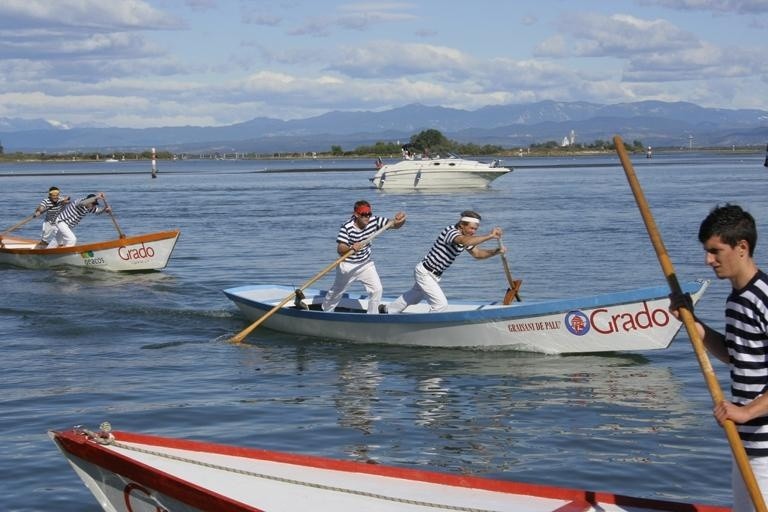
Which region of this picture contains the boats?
[47,421,734,512]
[368,147,514,188]
[0,229,180,272]
[223,279,709,356]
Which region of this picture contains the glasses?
[357,212,372,216]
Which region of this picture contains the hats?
[87,194,99,205]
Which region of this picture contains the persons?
[401,147,430,160]
[378,210,506,314]
[669,205,768,512]
[321,200,406,314]
[55,192,112,247]
[34,186,70,247]
